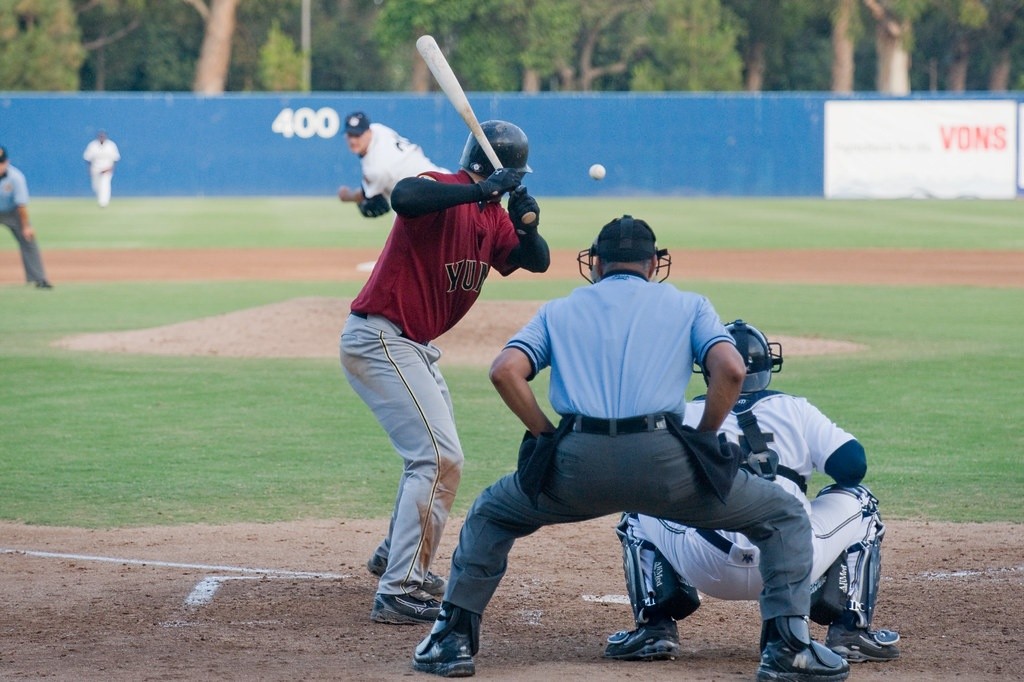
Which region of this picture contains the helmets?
[704,318,772,393]
[457,119,533,176]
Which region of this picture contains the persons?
[83,129,121,208]
[412,216,851,681]
[0,147,52,288]
[338,111,451,217]
[604,319,901,663]
[340,120,550,623]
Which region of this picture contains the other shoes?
[36,280,51,287]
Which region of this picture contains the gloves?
[480,166,521,202]
[505,187,541,244]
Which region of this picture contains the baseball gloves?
[357,193,390,217]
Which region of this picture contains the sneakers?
[367,552,449,595]
[757,616,850,680]
[603,612,679,660]
[371,590,441,623]
[825,622,900,661]
[412,602,482,677]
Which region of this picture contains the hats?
[345,111,370,137]
[595,214,656,264]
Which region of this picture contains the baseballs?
[588,163,606,180]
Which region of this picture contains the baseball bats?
[415,34,537,224]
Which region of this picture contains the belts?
[351,311,429,347]
[696,527,733,554]
[571,415,667,435]
